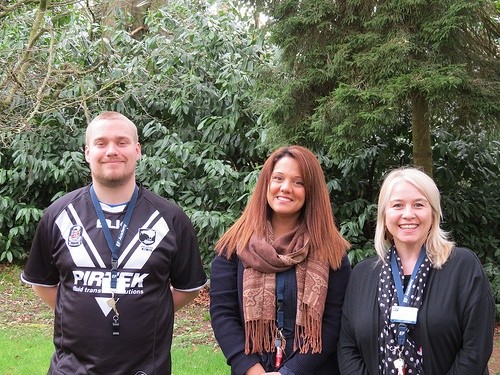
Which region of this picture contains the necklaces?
[90,184,139,336]
[276,272,286,368]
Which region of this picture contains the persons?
[337,167,494,375]
[20,111,207,375]
[211,146,351,375]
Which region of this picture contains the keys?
[107,298,119,316]
[393,357,405,375]
[275,338,282,354]
